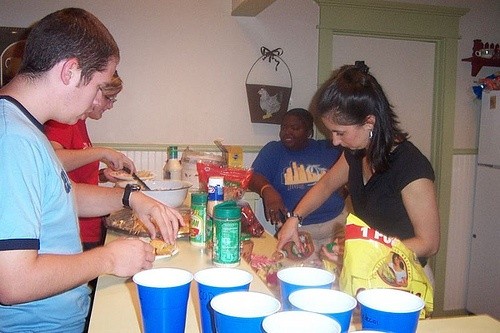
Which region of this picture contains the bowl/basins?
[114,179,193,207]
[106,168,156,181]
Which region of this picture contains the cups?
[261,311,343,333]
[357,288,425,333]
[277,267,335,311]
[132,267,193,333]
[194,267,253,333]
[209,290,281,333]
[288,288,358,333]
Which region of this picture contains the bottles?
[207,175,225,222]
[212,201,243,266]
[273,231,315,263]
[190,192,209,247]
[239,233,254,264]
[163,146,181,180]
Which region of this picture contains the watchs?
[123,183,141,211]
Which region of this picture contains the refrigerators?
[466,87,500,323]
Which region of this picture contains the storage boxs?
[214,140,243,168]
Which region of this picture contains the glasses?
[100,88,117,103]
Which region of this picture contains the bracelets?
[260,184,272,198]
[99,168,109,183]
[285,212,304,228]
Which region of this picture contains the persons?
[0,7,184,333]
[44,70,137,289]
[247,107,348,260]
[276,61,441,298]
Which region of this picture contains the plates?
[126,237,179,258]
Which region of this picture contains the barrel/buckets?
[179,151,224,193]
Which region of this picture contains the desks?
[88,189,500,333]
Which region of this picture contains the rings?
[271,209,274,214]
